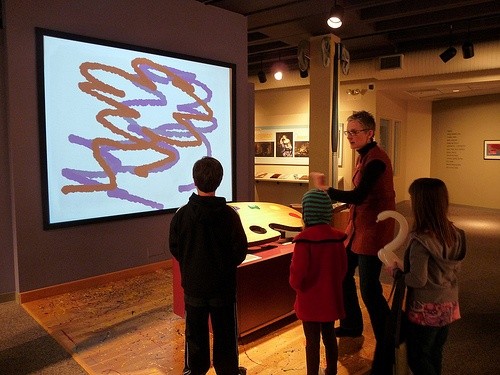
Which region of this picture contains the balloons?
[376,210,409,271]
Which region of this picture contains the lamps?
[326,1,344,29]
[462,22,475,59]
[439,25,457,63]
[257,57,268,83]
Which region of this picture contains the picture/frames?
[484,140,500,160]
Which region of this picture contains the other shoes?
[238,366,247,375]
[335,325,363,336]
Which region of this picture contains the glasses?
[343,128,369,136]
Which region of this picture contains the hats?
[301,188,334,224]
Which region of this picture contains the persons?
[309,110,395,341]
[169,157,248,375]
[383,178,466,375]
[289,189,348,375]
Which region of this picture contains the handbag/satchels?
[393,342,409,375]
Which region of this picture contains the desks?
[172,237,296,338]
